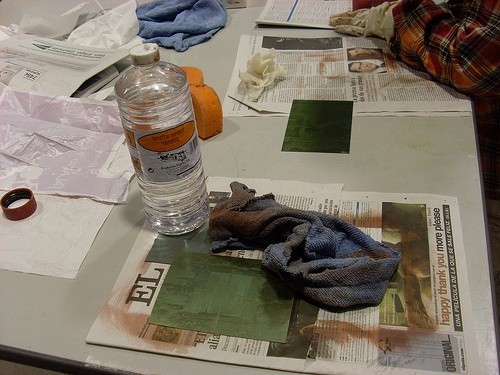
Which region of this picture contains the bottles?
[115,43,210,235]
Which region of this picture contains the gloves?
[328,0,398,45]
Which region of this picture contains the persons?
[347,48,383,59]
[347,59,389,75]
[329,0,500,323]
